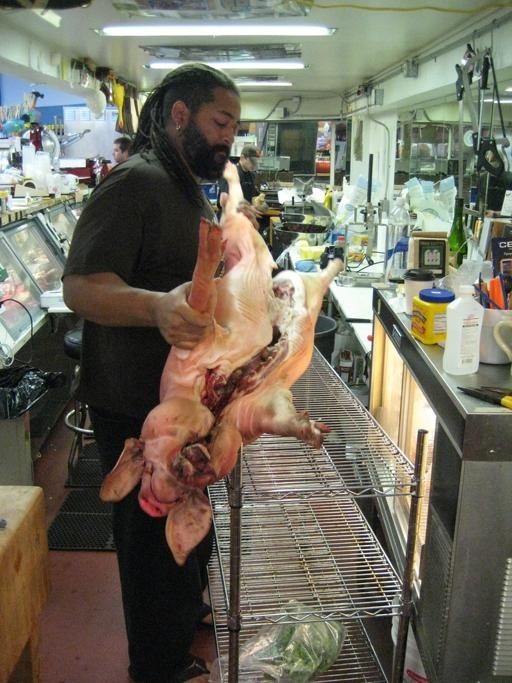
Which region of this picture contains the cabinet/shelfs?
[204,343,428,681]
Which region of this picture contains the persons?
[110,135,135,161]
[218,144,262,225]
[61,62,253,683]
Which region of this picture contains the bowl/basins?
[273,221,330,247]
[259,211,306,224]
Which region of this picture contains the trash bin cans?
[265,194,278,200]
[266,202,284,212]
[274,222,326,251]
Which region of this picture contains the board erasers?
[345,319,372,323]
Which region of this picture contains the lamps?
[88,19,333,86]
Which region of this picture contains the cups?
[481,308,510,364]
[401,267,434,316]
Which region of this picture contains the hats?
[242,144,262,163]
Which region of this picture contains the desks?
[323,272,374,409]
[0,483,54,682]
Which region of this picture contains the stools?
[60,326,102,487]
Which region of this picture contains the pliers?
[481,386,512,395]
[457,386,512,410]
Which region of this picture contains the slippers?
[128,657,211,683]
[197,603,214,627]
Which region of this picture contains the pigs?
[98,159,344,566]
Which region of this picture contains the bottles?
[444,284,481,371]
[388,197,408,282]
[412,288,452,345]
[445,197,469,270]
[468,186,479,209]
[334,235,345,244]
